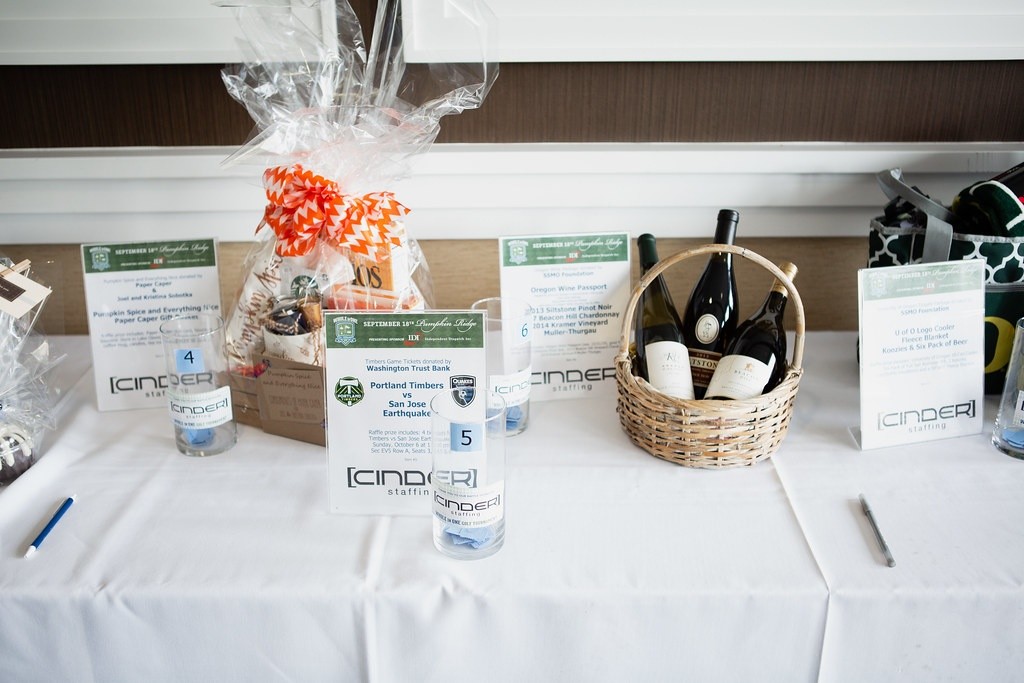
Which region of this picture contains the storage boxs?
[213,344,327,448]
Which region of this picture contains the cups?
[990,317,1024,461]
[159,312,238,458]
[428,387,507,560]
[472,296,534,438]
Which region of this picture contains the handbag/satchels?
[857,163,1023,395]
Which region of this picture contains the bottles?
[683,209,740,401]
[637,233,696,402]
[703,260,799,401]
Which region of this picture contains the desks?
[0,333,1023,683]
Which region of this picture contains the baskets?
[613,245,805,468]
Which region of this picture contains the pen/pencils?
[857,493,897,570]
[24,493,78,561]
